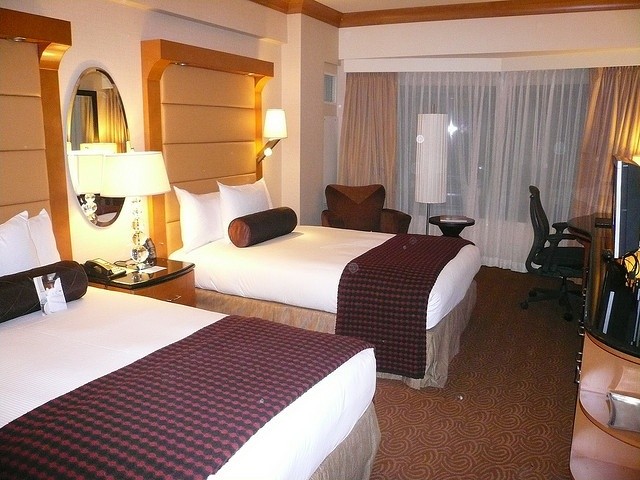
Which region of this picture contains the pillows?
[28,209,61,266]
[228,206,297,248]
[0,260,88,324]
[173,186,222,254]
[216,179,275,244]
[1,211,40,278]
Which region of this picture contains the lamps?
[257,108,289,164]
[79,141,118,153]
[416,115,447,236]
[69,150,113,230]
[97,147,174,280]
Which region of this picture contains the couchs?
[322,184,411,234]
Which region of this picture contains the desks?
[567,213,640,480]
[428,215,476,237]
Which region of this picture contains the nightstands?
[89,259,195,310]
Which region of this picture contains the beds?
[0,7,381,479]
[140,39,482,391]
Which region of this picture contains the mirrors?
[64,67,131,228]
[70,89,97,149]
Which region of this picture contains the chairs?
[520,186,591,321]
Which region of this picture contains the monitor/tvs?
[611,153,640,259]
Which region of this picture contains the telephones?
[80,258,126,290]
